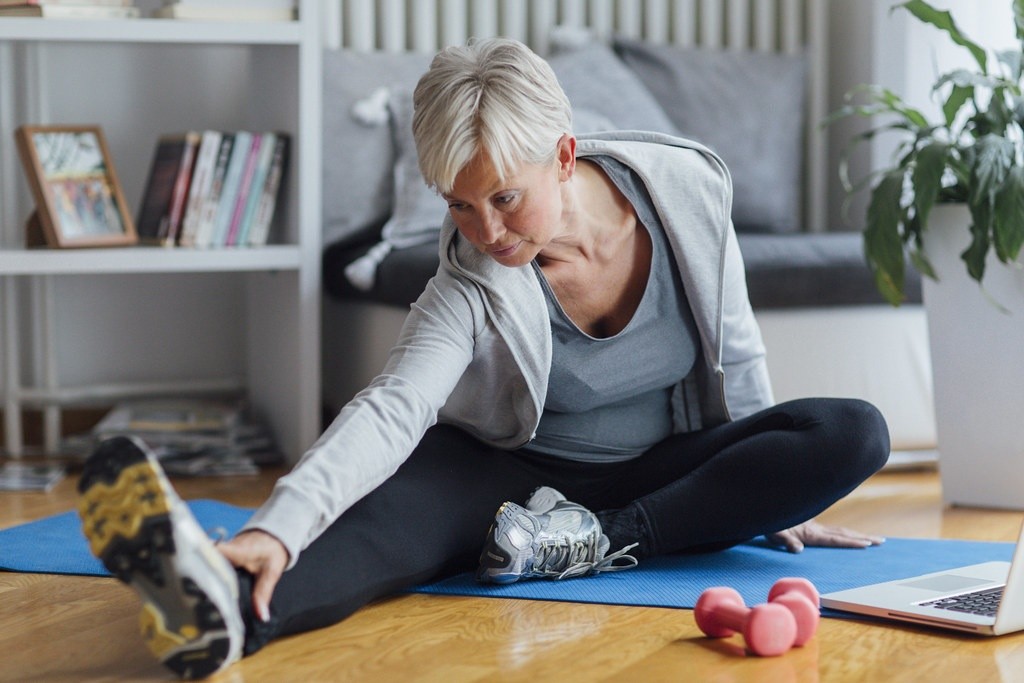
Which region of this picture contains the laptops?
[818,521,1024,635]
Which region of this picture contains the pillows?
[344,83,608,291]
[618,33,806,230]
[545,27,685,146]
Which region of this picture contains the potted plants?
[820,0,1024,510]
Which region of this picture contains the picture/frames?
[15,122,137,249]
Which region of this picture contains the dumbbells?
[693,586,798,656]
[768,576,819,647]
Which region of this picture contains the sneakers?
[77,431,249,683]
[476,483,641,585]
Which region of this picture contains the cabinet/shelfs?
[0,18,321,466]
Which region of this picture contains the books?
[136,130,289,248]
[98,397,274,476]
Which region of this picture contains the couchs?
[320,41,946,473]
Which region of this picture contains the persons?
[77,44,890,683]
